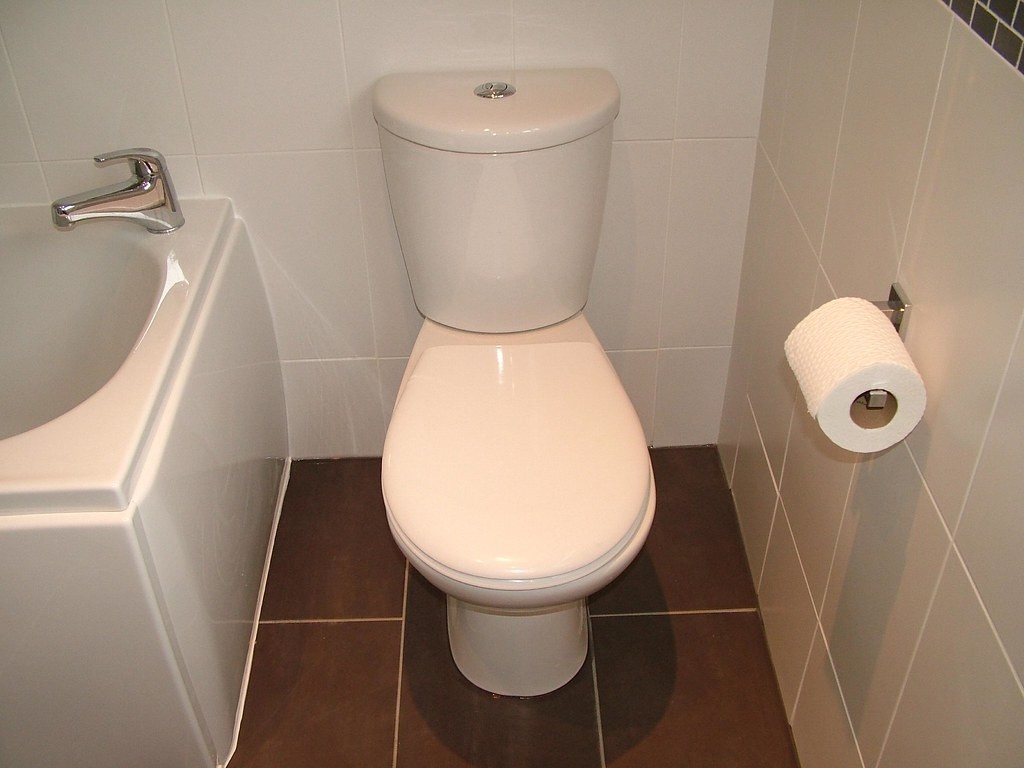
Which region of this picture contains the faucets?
[50,147,184,235]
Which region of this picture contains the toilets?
[373,67,658,697]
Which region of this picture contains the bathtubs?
[0,195,290,767]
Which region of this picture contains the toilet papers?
[783,295,927,454]
